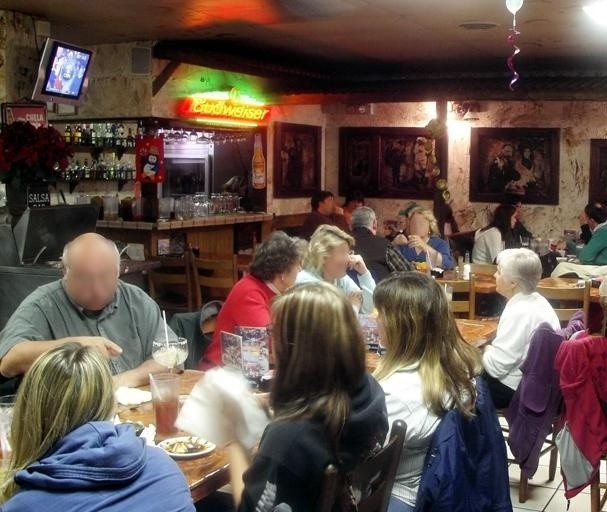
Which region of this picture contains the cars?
[158,192,241,221]
[148,374,181,435]
[518,233,547,253]
[440,284,453,308]
[74,195,139,222]
[0,394,17,473]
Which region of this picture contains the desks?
[23,251,161,289]
[537,277,599,309]
[3,314,500,512]
[436,264,499,293]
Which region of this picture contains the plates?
[45,141,138,185]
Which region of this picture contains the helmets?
[156,436,216,459]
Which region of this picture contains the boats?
[130,45,152,76]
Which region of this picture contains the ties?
[31,38,96,107]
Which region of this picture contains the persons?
[481,247,560,411]
[303,194,453,282]
[472,198,535,317]
[371,272,485,510]
[487,143,552,194]
[295,224,377,319]
[0,232,185,392]
[578,202,605,265]
[413,137,431,183]
[0,341,197,512]
[203,230,301,369]
[290,237,309,268]
[228,282,389,510]
[391,146,403,185]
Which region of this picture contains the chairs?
[495,311,585,503]
[535,280,590,329]
[436,273,475,319]
[148,250,191,319]
[191,253,238,311]
[562,336,606,512]
[446,228,605,275]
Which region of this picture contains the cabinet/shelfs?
[250,134,266,190]
[462,251,471,281]
[63,125,135,148]
[61,160,132,181]
[454,266,460,280]
[45,141,138,185]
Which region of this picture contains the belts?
[159,127,246,147]
[151,337,187,374]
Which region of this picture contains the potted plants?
[271,118,323,200]
[588,137,607,207]
[468,125,562,207]
[337,124,449,200]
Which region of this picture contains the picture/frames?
[588,137,607,207]
[337,124,449,200]
[271,118,323,200]
[468,125,562,207]
